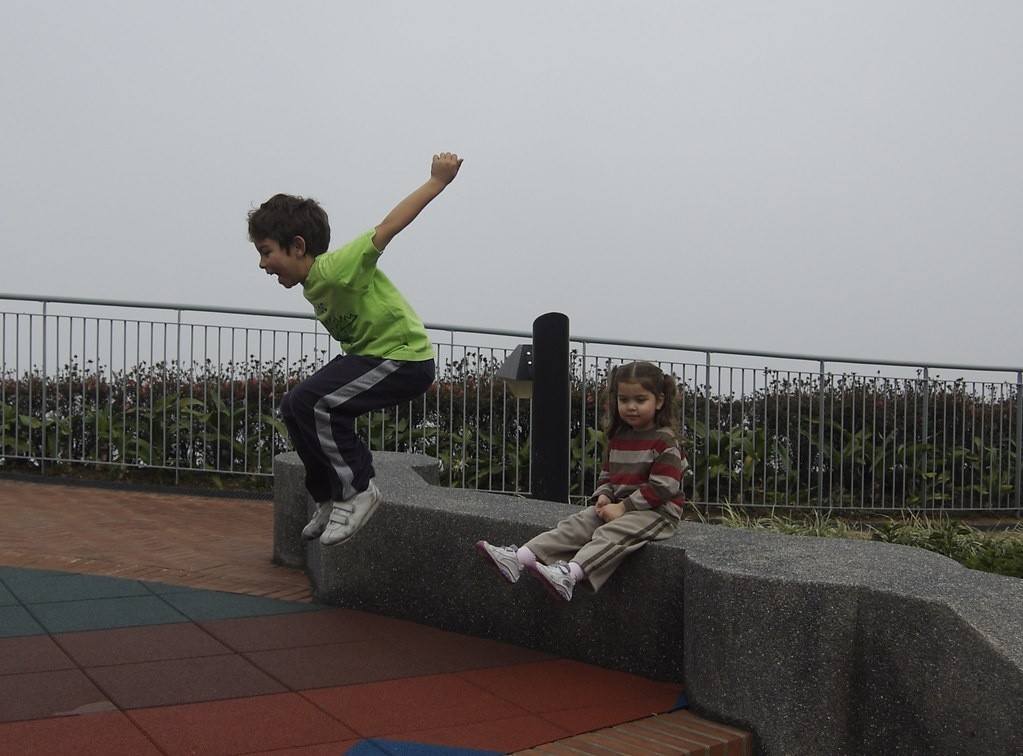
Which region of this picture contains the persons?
[475,361,694,603]
[246,152,464,549]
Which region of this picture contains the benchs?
[271,450,1023,756]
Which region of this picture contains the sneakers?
[526,560,576,602]
[320,478,383,548]
[476,540,525,583]
[301,500,333,540]
